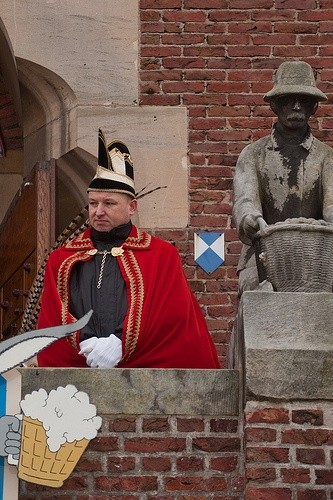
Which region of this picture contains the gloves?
[78,334,122,368]
[77,337,98,357]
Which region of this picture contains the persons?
[233,61,333,292]
[15,129,222,369]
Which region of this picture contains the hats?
[86,128,139,201]
